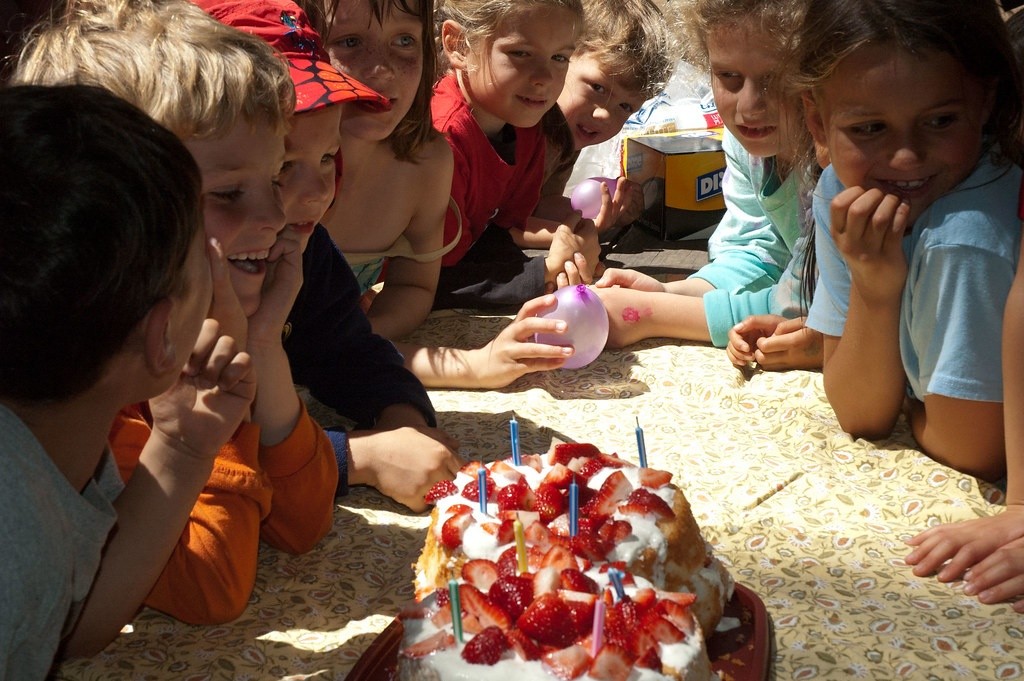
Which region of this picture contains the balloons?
[571,177,617,219]
[536,284,610,370]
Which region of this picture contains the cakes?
[397,438,731,681]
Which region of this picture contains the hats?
[191,0,392,115]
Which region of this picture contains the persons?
[0,0,670,681]
[551,0,815,348]
[722,0,1024,483]
[903,256,1024,615]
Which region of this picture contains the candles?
[448,570,463,645]
[568,475,580,536]
[510,410,519,465]
[478,460,488,511]
[591,588,606,653]
[514,513,528,572]
[635,415,648,467]
[604,557,623,597]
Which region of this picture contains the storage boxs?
[622,127,728,242]
